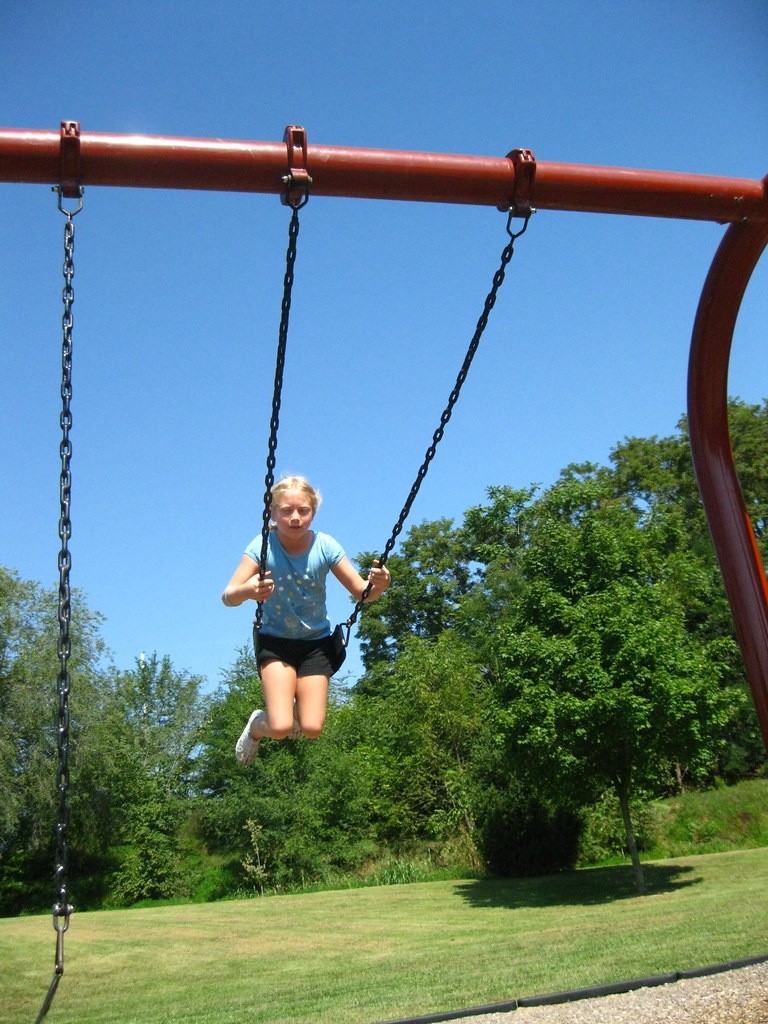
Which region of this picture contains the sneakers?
[289,697,302,734]
[236,710,266,768]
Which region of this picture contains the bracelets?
[224,590,242,607]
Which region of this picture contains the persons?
[222,475,390,766]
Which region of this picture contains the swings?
[244,120,544,683]
[30,115,87,1024]
